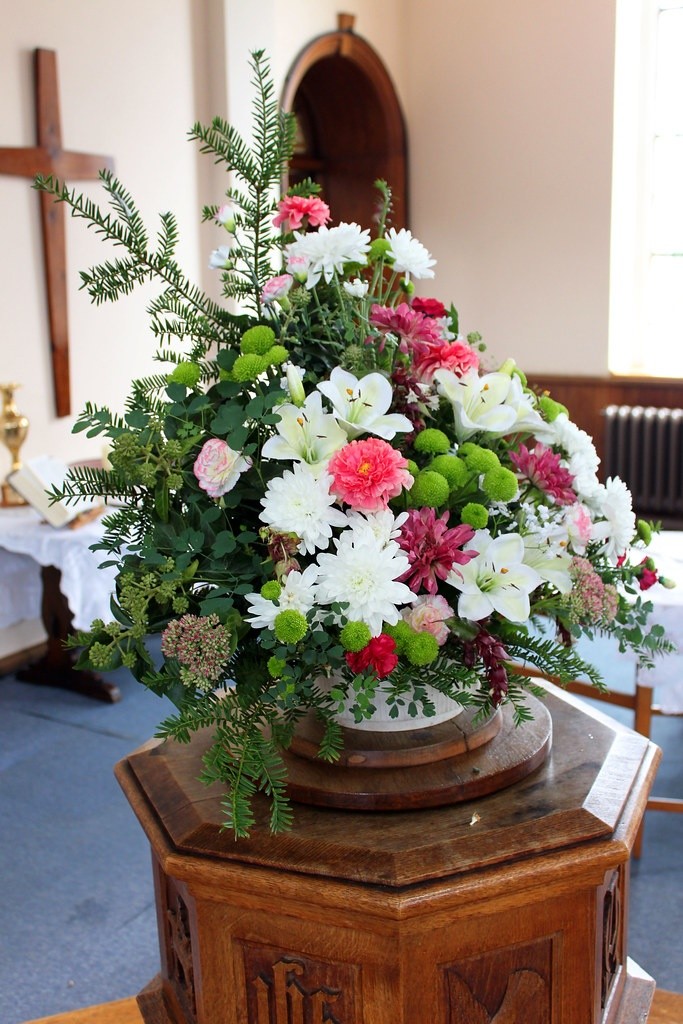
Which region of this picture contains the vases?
[331,661,471,732]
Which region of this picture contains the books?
[6,452,99,531]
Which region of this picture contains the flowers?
[30,44,673,842]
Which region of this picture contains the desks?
[0,499,140,705]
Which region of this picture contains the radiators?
[606,405,683,521]
